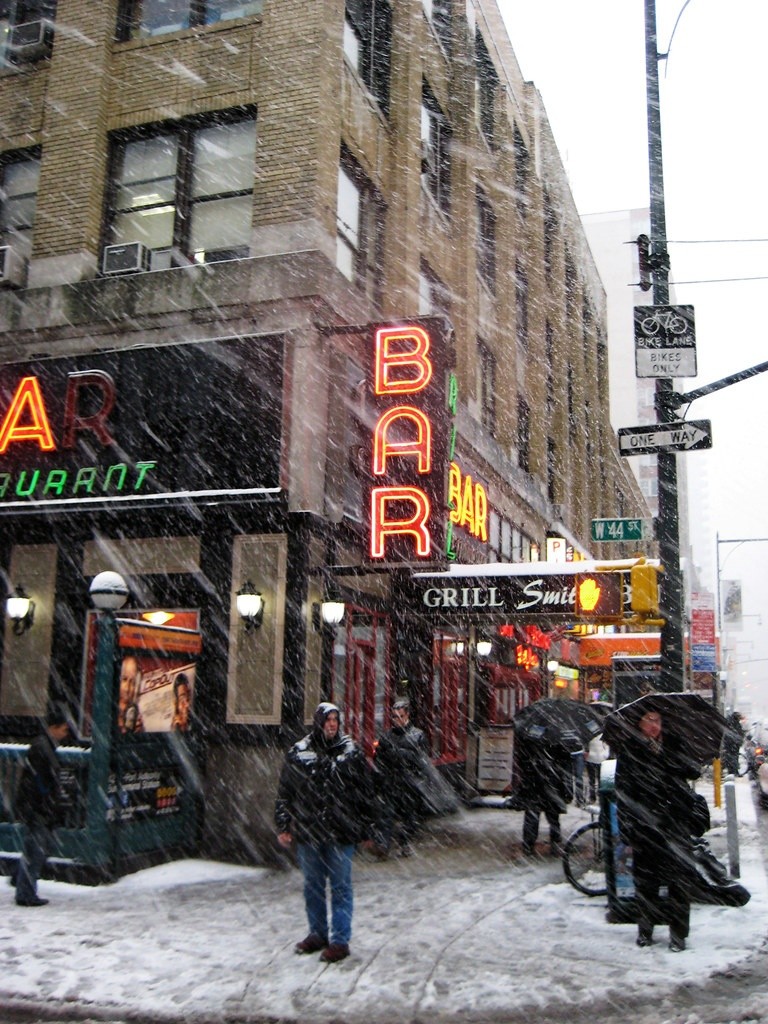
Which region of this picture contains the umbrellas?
[589,700,616,715]
[505,698,605,744]
[599,690,729,778]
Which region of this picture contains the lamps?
[476,634,491,661]
[545,656,559,676]
[237,579,263,632]
[88,568,129,622]
[320,588,345,635]
[6,584,32,636]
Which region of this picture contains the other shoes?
[637,930,652,944]
[17,898,48,906]
[667,937,685,951]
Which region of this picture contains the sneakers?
[296,934,329,954]
[320,944,348,962]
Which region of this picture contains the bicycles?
[562,804,631,896]
[739,753,750,777]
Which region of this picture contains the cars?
[742,717,768,810]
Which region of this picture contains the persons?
[118,656,145,734]
[586,734,610,804]
[614,705,694,952]
[10,711,67,907]
[510,732,574,858]
[725,711,746,777]
[368,702,426,862]
[171,673,192,731]
[274,702,372,963]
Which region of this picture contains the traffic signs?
[618,418,713,457]
[590,519,643,543]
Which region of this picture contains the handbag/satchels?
[690,796,711,836]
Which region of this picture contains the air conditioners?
[101,242,151,277]
[9,19,54,56]
[0,244,27,290]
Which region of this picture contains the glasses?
[393,714,406,719]
